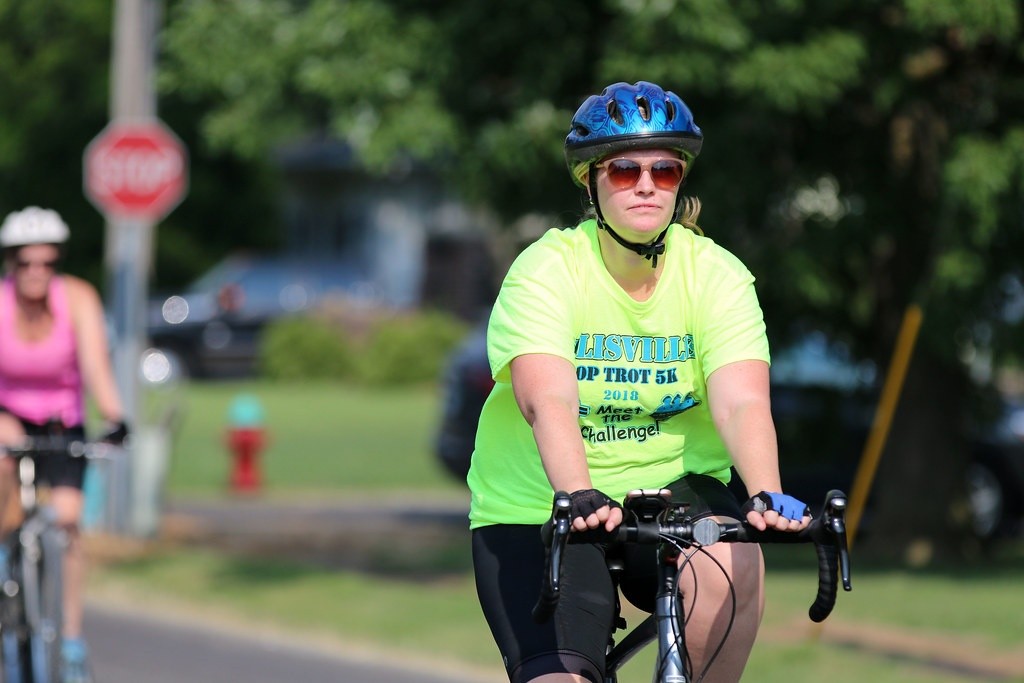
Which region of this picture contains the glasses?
[13,259,59,270]
[597,157,687,192]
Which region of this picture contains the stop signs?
[82,121,189,225]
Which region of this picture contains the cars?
[107,255,405,381]
[433,300,1023,548]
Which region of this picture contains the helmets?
[0,206,71,249]
[564,81,704,189]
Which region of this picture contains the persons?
[464,81,815,683]
[0,204,139,683]
[431,287,500,483]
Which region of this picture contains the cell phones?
[626,489,672,503]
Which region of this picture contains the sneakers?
[59,637,92,683]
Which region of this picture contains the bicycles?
[1,414,130,682]
[530,488,851,683]
